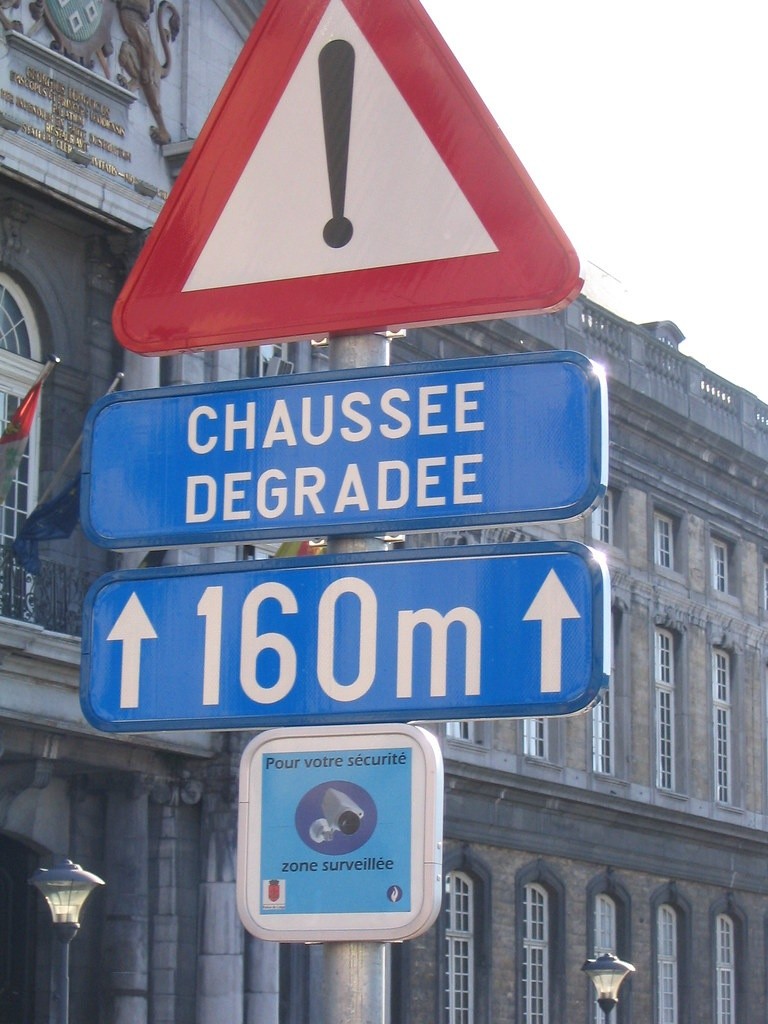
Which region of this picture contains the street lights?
[581,953,636,1024]
[26,855,110,1024]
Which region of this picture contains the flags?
[0,380,48,508]
[11,470,82,574]
